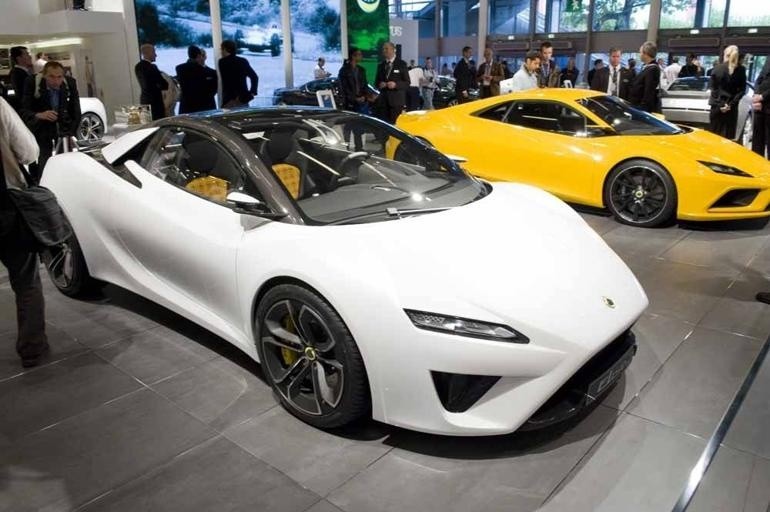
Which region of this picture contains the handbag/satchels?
[7,181,72,249]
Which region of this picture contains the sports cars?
[232,22,296,58]
[20,104,649,438]
[381,87,770,227]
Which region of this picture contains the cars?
[75,98,109,147]
[408,76,480,111]
[660,75,754,151]
[272,78,379,109]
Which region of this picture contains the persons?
[1,46,81,182]
[588,42,770,159]
[0,93,49,369]
[407,40,579,115]
[315,42,410,155]
[134,41,259,119]
[756,292,770,304]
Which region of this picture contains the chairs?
[168,128,238,199]
[253,119,316,195]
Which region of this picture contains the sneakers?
[15,336,49,368]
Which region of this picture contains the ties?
[384,60,393,78]
[609,67,620,99]
[486,66,490,75]
